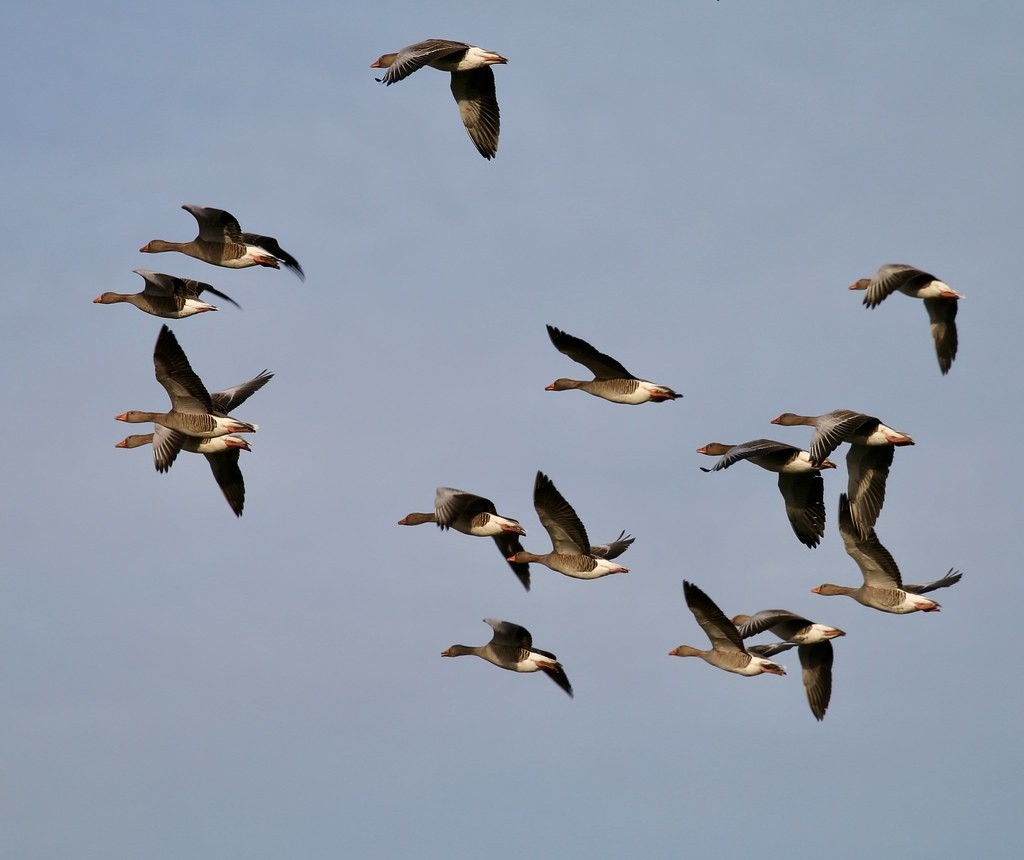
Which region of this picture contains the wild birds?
[667,580,846,723]
[695,408,915,550]
[441,616,573,698]
[397,470,636,592]
[811,493,963,615]
[545,324,684,406]
[847,264,966,377]
[93,205,309,516]
[370,38,510,161]
